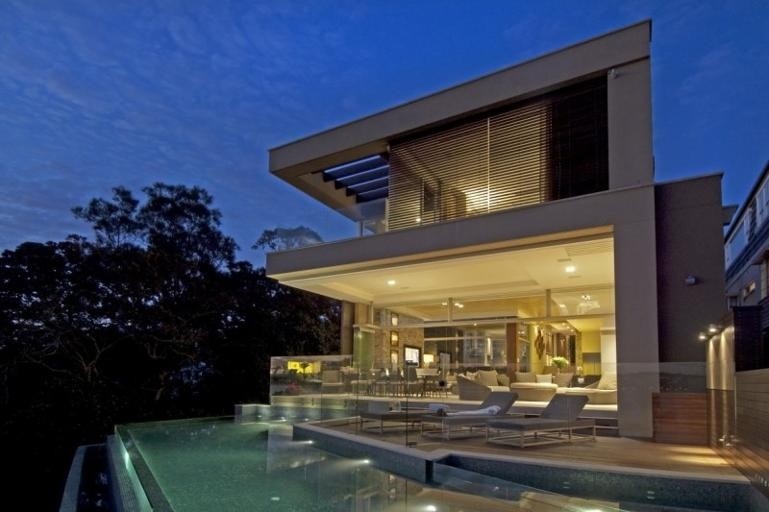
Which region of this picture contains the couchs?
[457,371,512,399]
[510,371,574,400]
[556,385,617,403]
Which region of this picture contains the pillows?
[515,371,536,382]
[497,374,510,385]
[595,371,617,390]
[535,374,553,384]
[476,370,499,387]
[465,371,477,380]
[553,373,573,386]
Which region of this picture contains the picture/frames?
[390,349,399,365]
[390,331,399,347]
[390,311,400,328]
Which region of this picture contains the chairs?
[483,393,597,448]
[366,367,448,398]
[420,392,524,441]
[361,407,456,434]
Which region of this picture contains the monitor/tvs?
[403,344,422,366]
